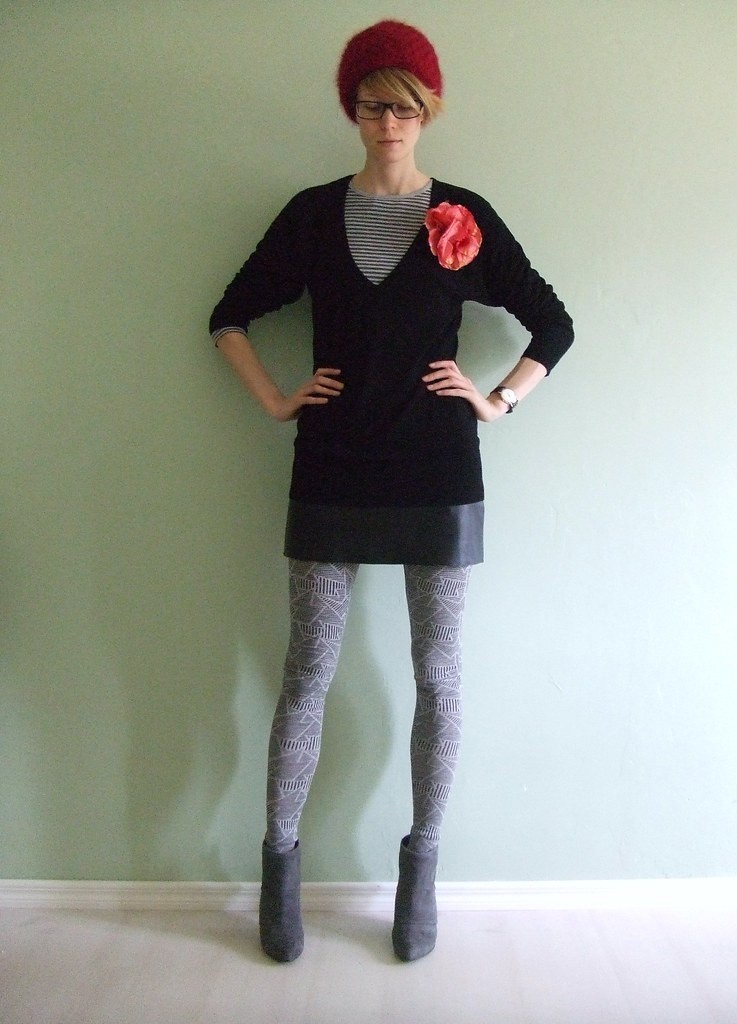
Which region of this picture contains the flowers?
[423,201,483,270]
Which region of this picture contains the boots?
[392,835,438,961]
[259,838,305,962]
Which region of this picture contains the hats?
[336,21,441,122]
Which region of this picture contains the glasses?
[354,100,422,120]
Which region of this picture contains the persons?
[211,20,574,962]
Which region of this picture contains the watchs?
[489,386,519,414]
